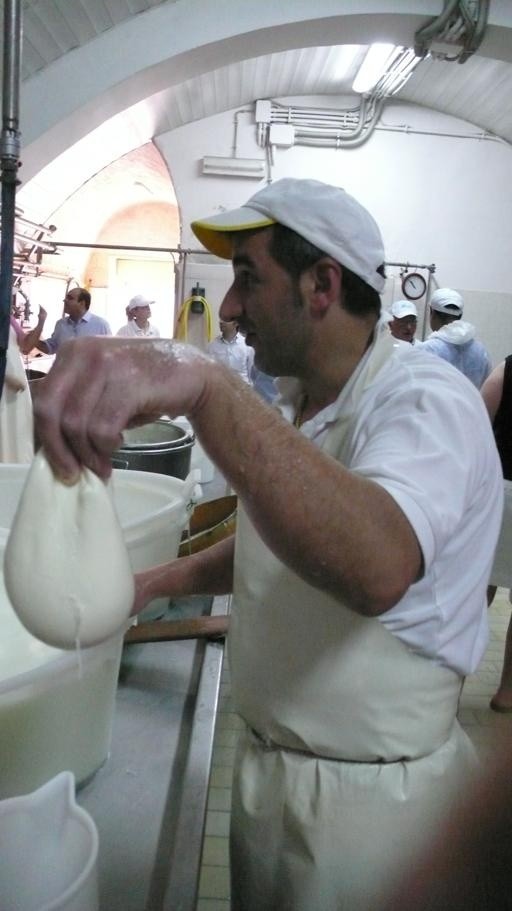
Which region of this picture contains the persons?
[8,286,159,356]
[388,286,510,714]
[33,178,512,910]
[210,320,279,401]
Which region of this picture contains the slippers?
[490,699,510,713]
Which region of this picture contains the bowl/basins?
[116,418,195,480]
[2,463,195,805]
[180,495,239,554]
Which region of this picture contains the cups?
[2,772,102,910]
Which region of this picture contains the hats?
[128,296,155,312]
[191,179,386,294]
[431,287,464,316]
[391,300,418,319]
